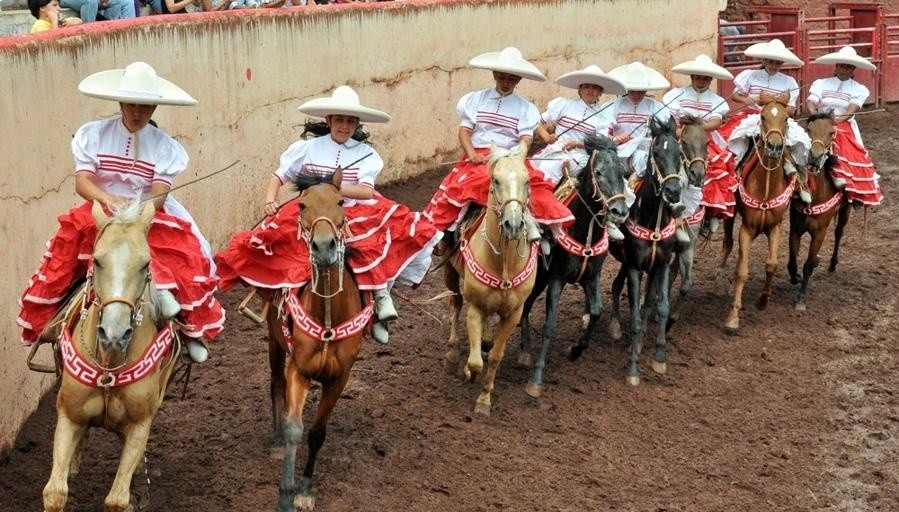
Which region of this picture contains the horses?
[581,115,682,389]
[442,137,540,416]
[786,109,851,310]
[696,89,795,335]
[643,113,712,340]
[516,134,630,397]
[264,164,366,512]
[42,197,176,512]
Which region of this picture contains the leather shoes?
[607,221,625,242]
[832,176,847,188]
[371,295,399,345]
[524,211,551,256]
[709,216,720,234]
[157,287,209,364]
[784,162,812,204]
[671,202,690,243]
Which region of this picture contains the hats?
[77,61,198,106]
[607,62,670,91]
[554,65,627,95]
[743,38,804,66]
[815,46,877,71]
[670,53,734,79]
[298,85,392,124]
[470,47,546,82]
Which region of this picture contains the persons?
[526,63,627,242]
[600,60,693,246]
[803,43,886,213]
[662,53,739,234]
[14,58,228,366]
[210,85,446,344]
[719,37,814,209]
[418,45,576,265]
[24,0,399,40]
